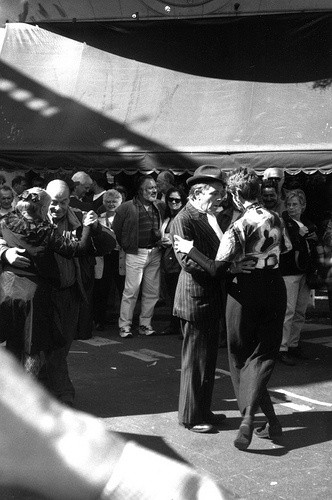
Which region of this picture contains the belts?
[138,243,158,249]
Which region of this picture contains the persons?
[113,177,163,339]
[171,164,256,432]
[261,182,282,211]
[281,186,319,365]
[174,168,291,450]
[0,169,187,406]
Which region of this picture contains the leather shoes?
[205,413,226,422]
[255,423,282,440]
[187,423,213,433]
[234,425,251,451]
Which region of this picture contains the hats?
[186,164,228,186]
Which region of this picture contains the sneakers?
[119,328,132,338]
[140,328,155,335]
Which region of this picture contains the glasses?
[268,177,285,182]
[167,196,183,203]
